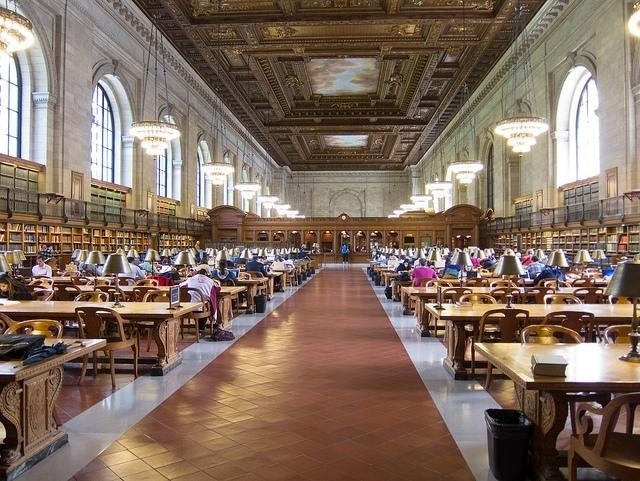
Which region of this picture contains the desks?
[473,343,640,481]
[0,338,107,481]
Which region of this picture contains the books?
[492,222,639,257]
[0,220,195,254]
[532,354,568,378]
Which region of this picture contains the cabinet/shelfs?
[490,218,640,269]
[0,218,202,256]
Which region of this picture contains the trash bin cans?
[296,266,316,285]
[366,265,383,285]
[253,295,267,314]
[484,409,533,481]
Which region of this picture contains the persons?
[0,274,32,301]
[370,245,616,288]
[32,256,52,278]
[341,241,350,264]
[76,241,293,339]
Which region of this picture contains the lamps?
[257,93,306,219]
[130,0,182,156]
[425,30,453,198]
[0,0,35,53]
[387,88,432,218]
[235,55,261,199]
[494,0,550,153]
[201,0,234,186]
[451,0,484,185]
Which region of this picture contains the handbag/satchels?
[0,334,45,361]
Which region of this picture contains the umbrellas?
[12,338,86,369]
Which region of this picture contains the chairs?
[521,323,611,435]
[370,246,640,389]
[567,392,640,481]
[3,318,64,426]
